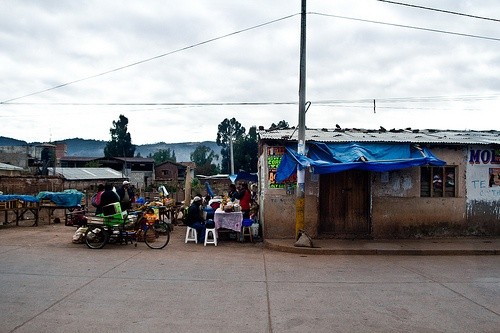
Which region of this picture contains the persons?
[92,181,255,243]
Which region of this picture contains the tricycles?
[84,209,170,250]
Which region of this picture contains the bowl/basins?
[240,219,253,227]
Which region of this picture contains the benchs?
[65,211,84,225]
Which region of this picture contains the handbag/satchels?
[294,231,314,247]
[205,219,215,229]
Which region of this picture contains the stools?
[185,226,197,244]
[241,226,253,243]
[204,227,217,247]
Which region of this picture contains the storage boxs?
[243,219,252,226]
[103,210,129,227]
[102,202,122,216]
[5,193,86,209]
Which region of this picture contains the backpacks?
[92,191,105,207]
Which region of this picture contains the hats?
[194,196,201,201]
[123,181,130,185]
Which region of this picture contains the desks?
[158,209,178,227]
[0,206,39,228]
[214,208,244,242]
[40,206,85,226]
